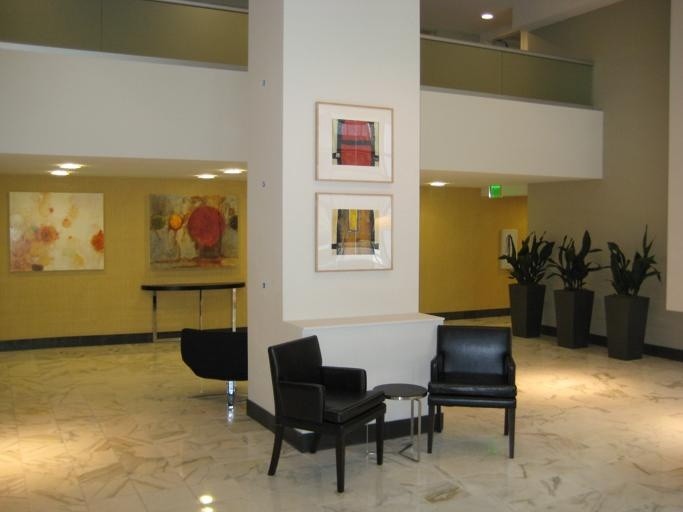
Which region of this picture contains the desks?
[142,282,245,344]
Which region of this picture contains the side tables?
[367,383,427,462]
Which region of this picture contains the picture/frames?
[315,192,393,272]
[315,102,394,183]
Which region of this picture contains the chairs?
[428,325,517,459]
[268,335,387,493]
[180,328,247,406]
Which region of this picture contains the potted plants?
[497,230,555,338]
[603,224,661,360]
[546,230,603,348]
